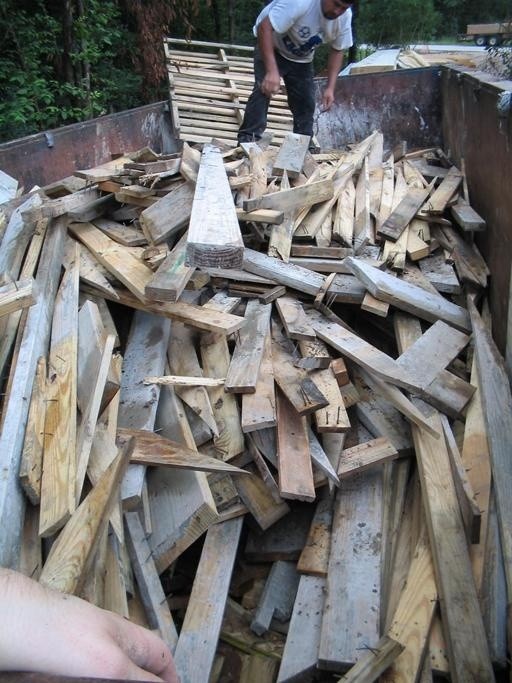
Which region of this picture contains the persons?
[1,561,183,682]
[237,0,355,146]
